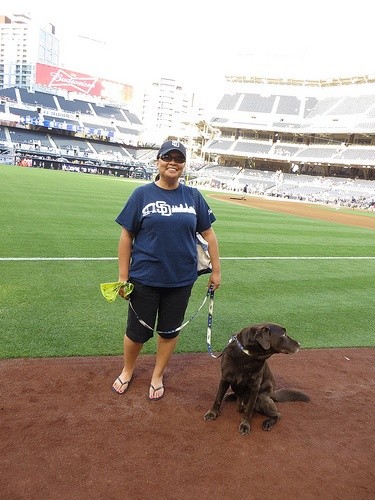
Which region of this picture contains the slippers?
[149,383,164,401]
[112,376,128,395]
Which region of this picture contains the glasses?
[158,154,186,164]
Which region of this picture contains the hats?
[157,140,187,161]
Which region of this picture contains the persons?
[91,134,109,141]
[333,196,375,212]
[111,140,221,402]
[109,170,111,176]
[241,184,248,200]
[99,169,104,175]
[115,171,118,176]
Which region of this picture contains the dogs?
[204,322,310,437]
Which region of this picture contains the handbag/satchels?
[195,231,212,275]
